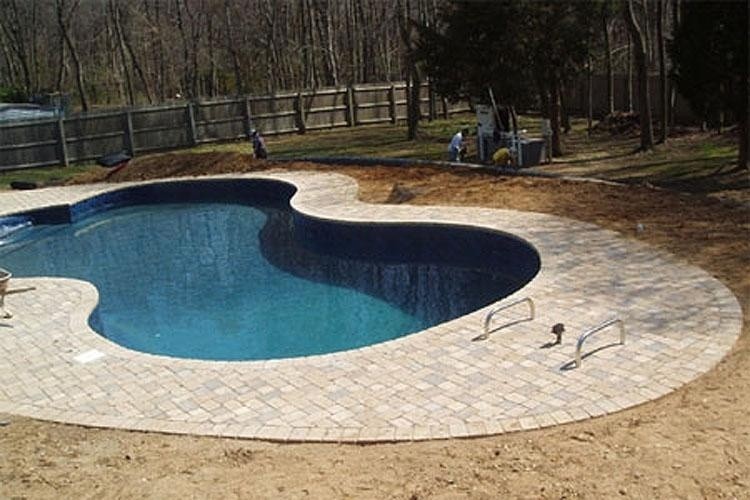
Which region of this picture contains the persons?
[250,130,267,160]
[541,111,554,163]
[448,128,468,162]
[491,146,518,182]
